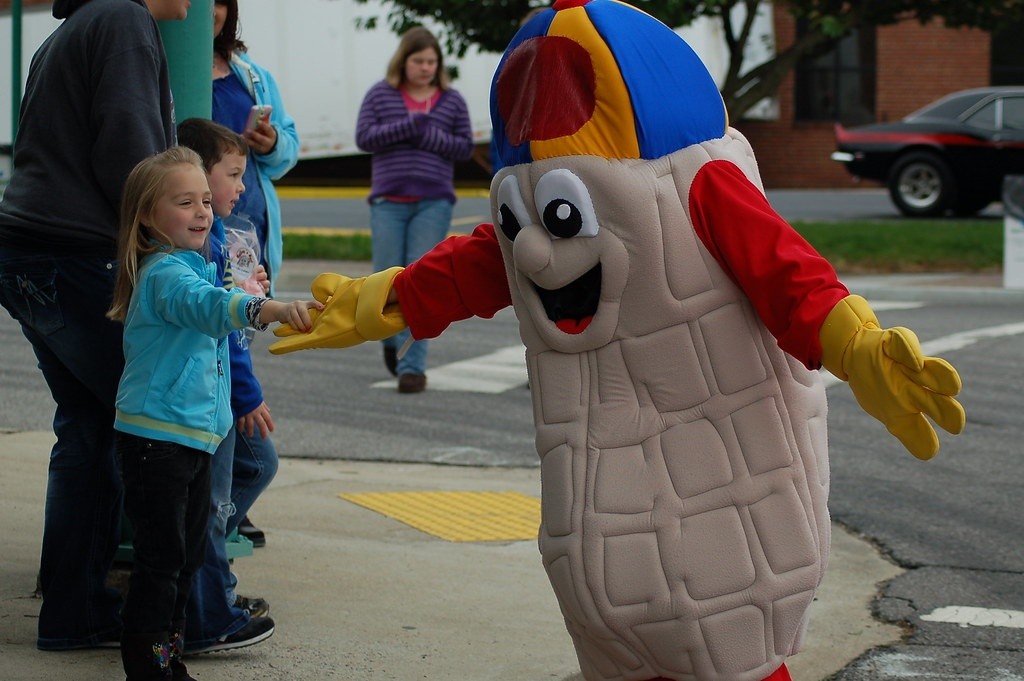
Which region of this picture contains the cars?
[831,88,1024,219]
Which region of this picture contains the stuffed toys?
[268,0,966,681]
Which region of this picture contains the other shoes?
[397,373,426,393]
[238,519,268,547]
[384,346,398,376]
[234,593,269,617]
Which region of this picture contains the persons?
[106,142,324,681]
[177,117,275,653]
[354,28,475,394]
[211,0,301,302]
[1,0,274,654]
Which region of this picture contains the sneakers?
[178,614,277,656]
[38,601,122,651]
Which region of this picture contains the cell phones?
[244,104,273,133]
